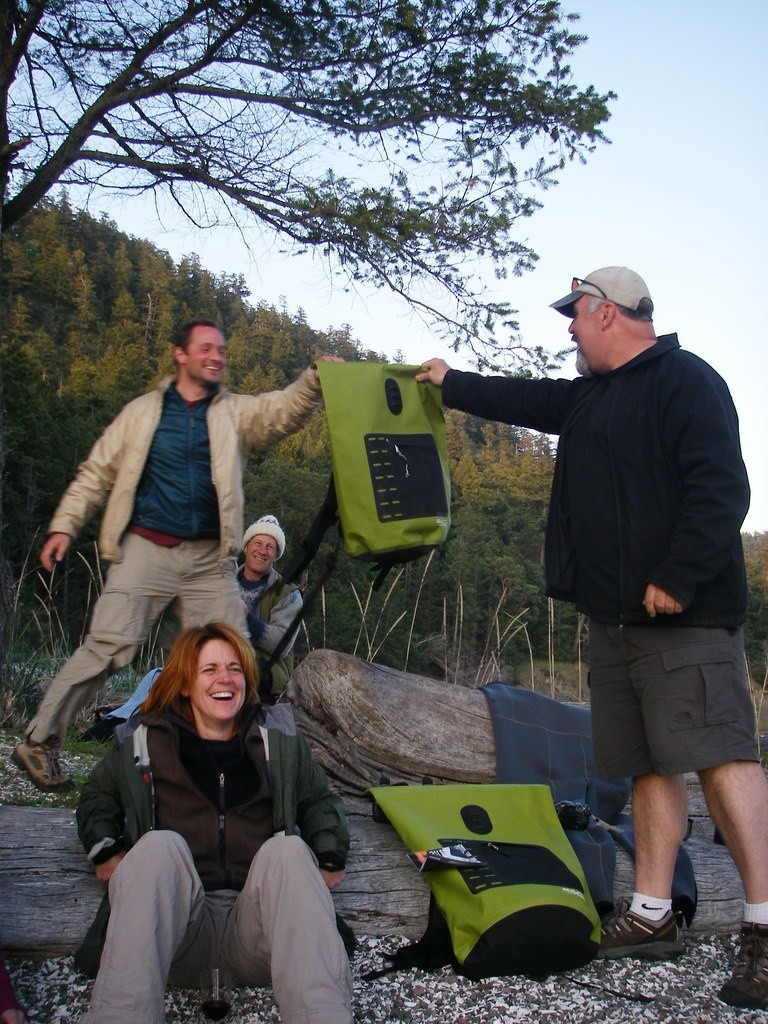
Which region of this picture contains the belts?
[127,524,183,548]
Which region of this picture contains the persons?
[11,321,347,792]
[416,267,768,1011]
[77,622,358,1024]
[0,950,30,1024]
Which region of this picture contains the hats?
[242,514,286,561]
[548,266,654,318]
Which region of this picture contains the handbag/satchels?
[308,359,453,561]
[362,783,603,978]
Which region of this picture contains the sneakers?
[596,895,687,961]
[11,740,75,793]
[718,921,768,1011]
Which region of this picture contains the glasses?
[571,277,606,299]
